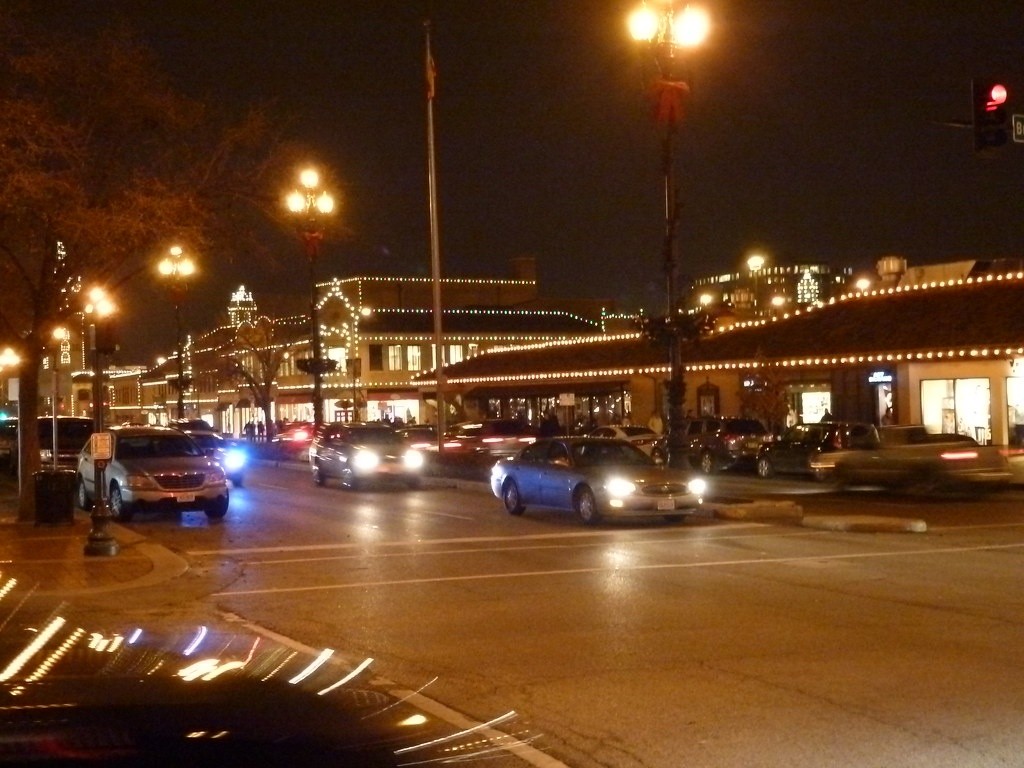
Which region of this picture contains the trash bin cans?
[31,470,76,527]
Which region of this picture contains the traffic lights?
[971,69,1009,152]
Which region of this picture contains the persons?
[378,414,417,429]
[882,408,895,425]
[244,418,297,445]
[541,410,694,436]
[821,409,833,422]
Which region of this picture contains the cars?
[387,422,441,454]
[806,423,1008,506]
[0,417,19,463]
[490,438,704,526]
[439,418,540,465]
[271,421,340,462]
[76,427,231,522]
[586,426,659,465]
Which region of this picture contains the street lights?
[74,281,114,432]
[160,245,199,419]
[40,323,67,425]
[0,346,20,419]
[280,166,338,483]
[625,3,708,479]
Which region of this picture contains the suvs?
[165,419,226,465]
[310,421,421,491]
[652,415,771,477]
[753,417,878,487]
[35,417,93,471]
